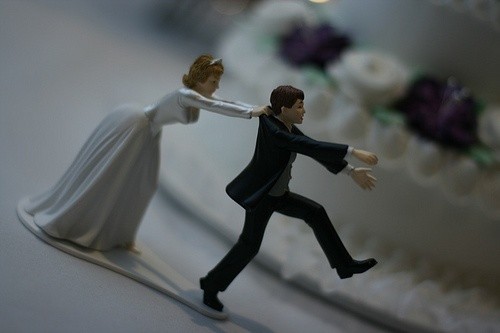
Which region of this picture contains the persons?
[199,85,378,312]
[25,53,275,255]
